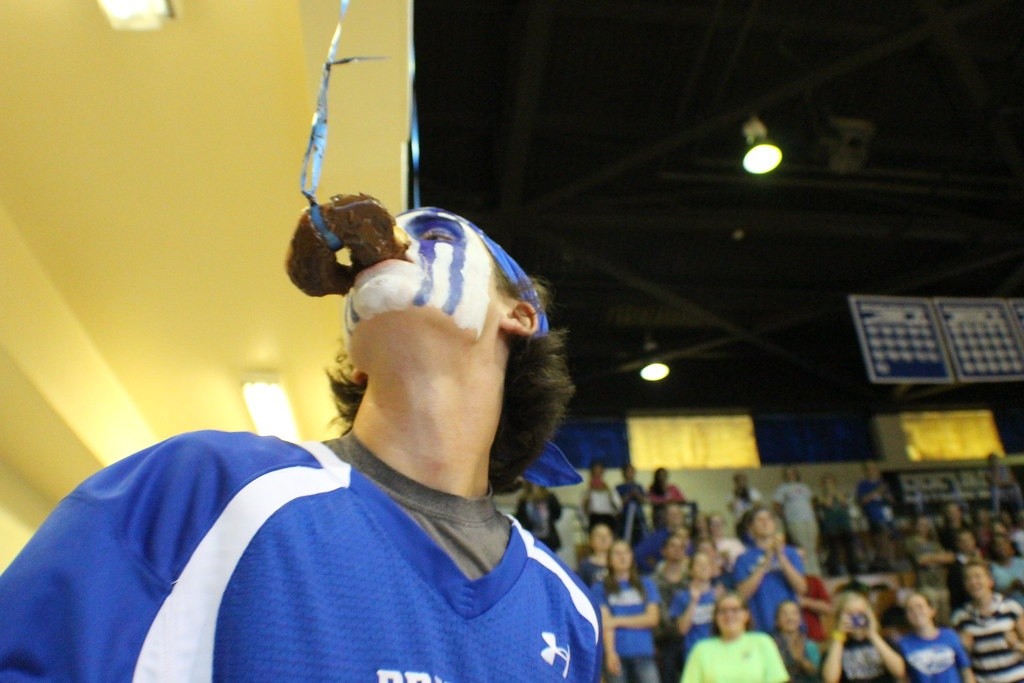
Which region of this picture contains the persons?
[509,452,1023,683]
[1,205,610,681]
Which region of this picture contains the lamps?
[639,342,670,382]
[741,116,783,176]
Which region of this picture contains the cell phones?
[848,615,868,626]
